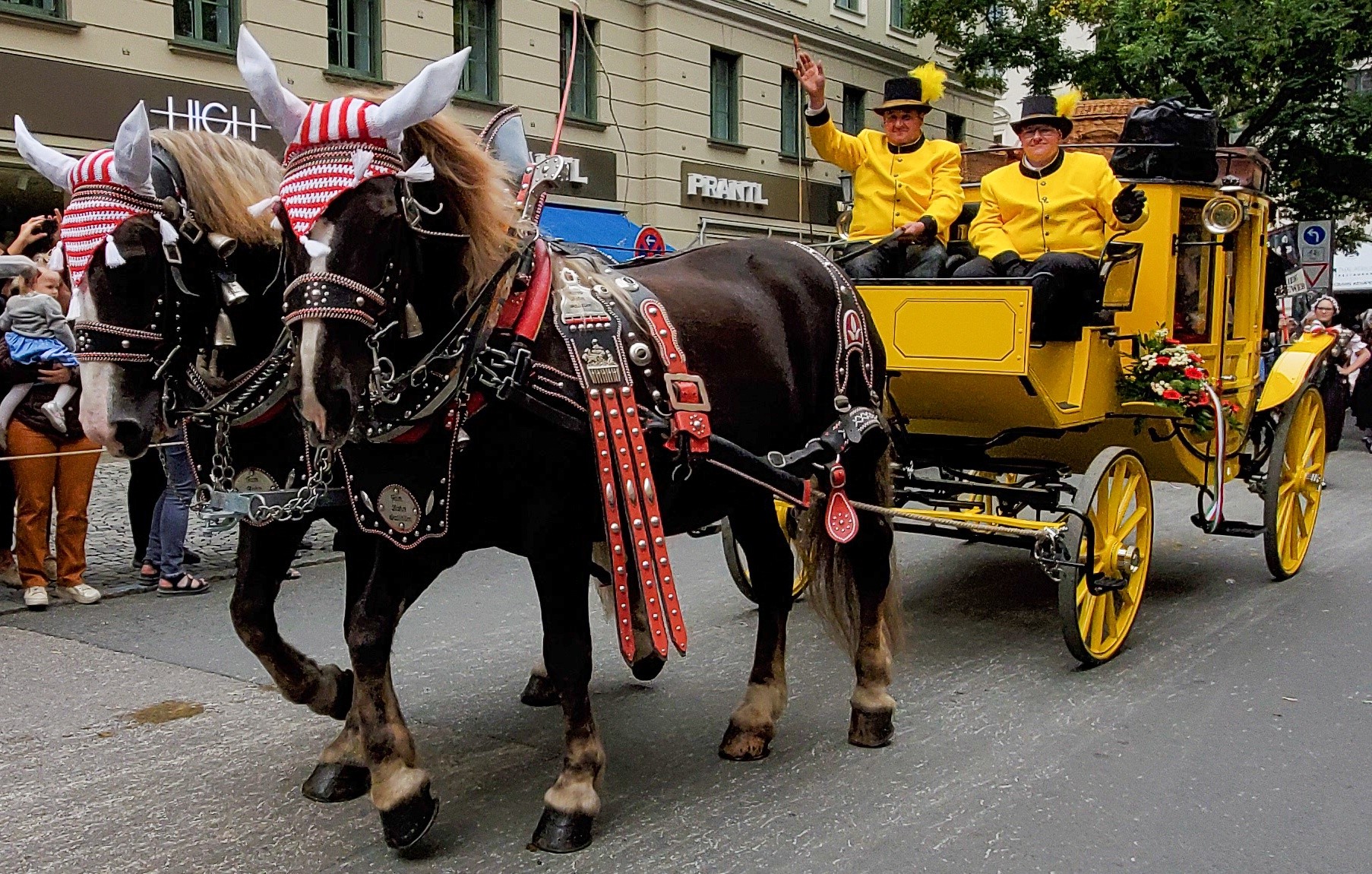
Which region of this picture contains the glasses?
[1316,306,1333,313]
[1018,126,1060,138]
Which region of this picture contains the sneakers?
[24,585,49,606]
[55,582,101,604]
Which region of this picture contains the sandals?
[155,570,209,597]
[136,560,160,586]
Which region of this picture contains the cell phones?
[41,220,58,233]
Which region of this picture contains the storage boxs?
[959,147,1007,183]
[1215,147,1271,193]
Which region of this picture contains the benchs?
[821,202,1123,418]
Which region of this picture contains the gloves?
[1114,182,1147,215]
[1002,259,1031,278]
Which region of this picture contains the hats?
[874,62,948,116]
[1310,295,1340,315]
[1010,90,1083,139]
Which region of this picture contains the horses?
[11,22,912,853]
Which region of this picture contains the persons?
[950,96,1149,338]
[1266,295,1372,488]
[791,50,966,284]
[0,210,314,608]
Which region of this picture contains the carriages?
[16,24,1334,851]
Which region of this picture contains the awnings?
[537,205,678,264]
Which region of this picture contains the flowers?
[1114,319,1250,437]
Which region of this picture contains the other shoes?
[0,423,8,450]
[0,550,23,585]
[1321,479,1327,489]
[132,550,144,567]
[182,545,201,564]
[1361,436,1372,454]
[42,553,59,581]
[41,401,67,433]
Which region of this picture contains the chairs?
[1173,271,1212,343]
